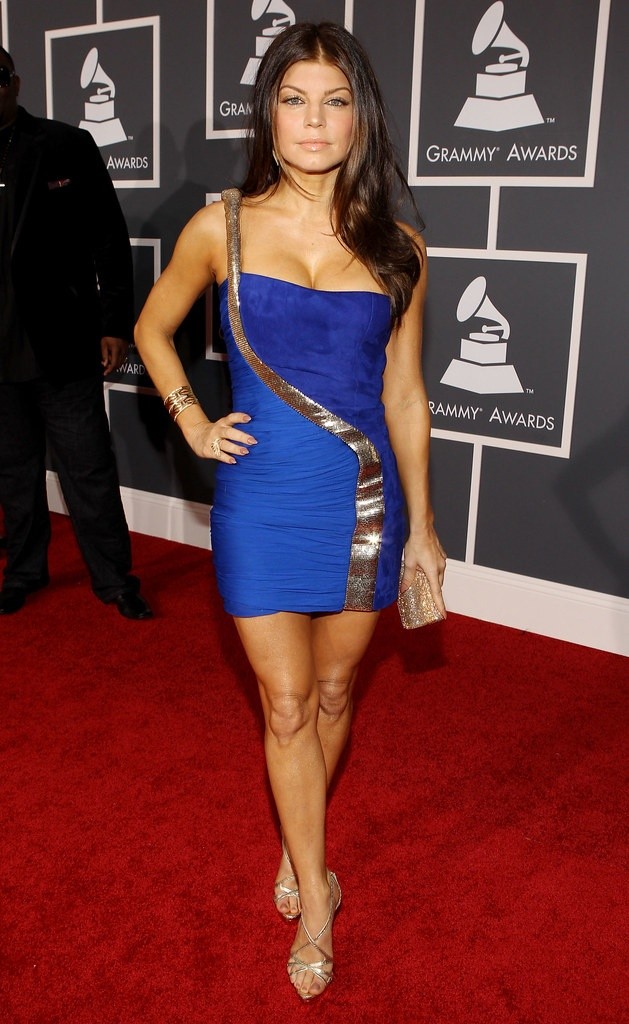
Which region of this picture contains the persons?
[0,44,152,620]
[134,22,448,1000]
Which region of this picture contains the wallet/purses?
[397,547,443,628]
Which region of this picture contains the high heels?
[287,870,342,1001]
[274,844,303,919]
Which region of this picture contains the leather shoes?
[95,577,153,620]
[0,574,47,614]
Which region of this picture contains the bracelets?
[163,385,199,423]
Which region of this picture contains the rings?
[211,438,225,457]
